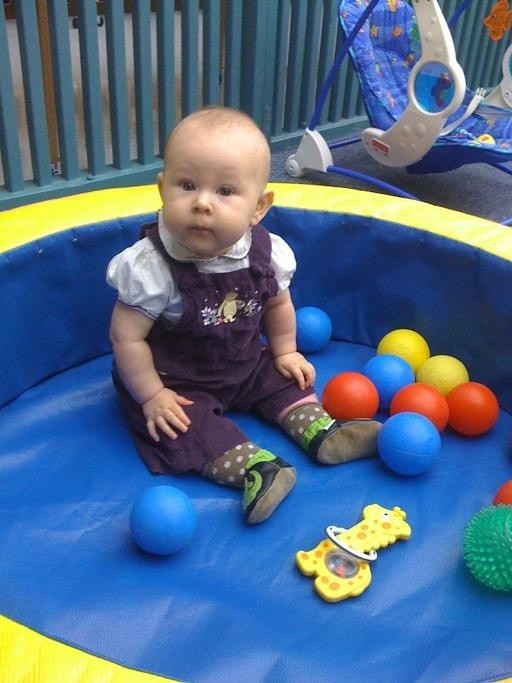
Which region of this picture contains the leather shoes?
[242,450,297,524]
[302,416,383,465]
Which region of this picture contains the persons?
[105,107,383,525]
